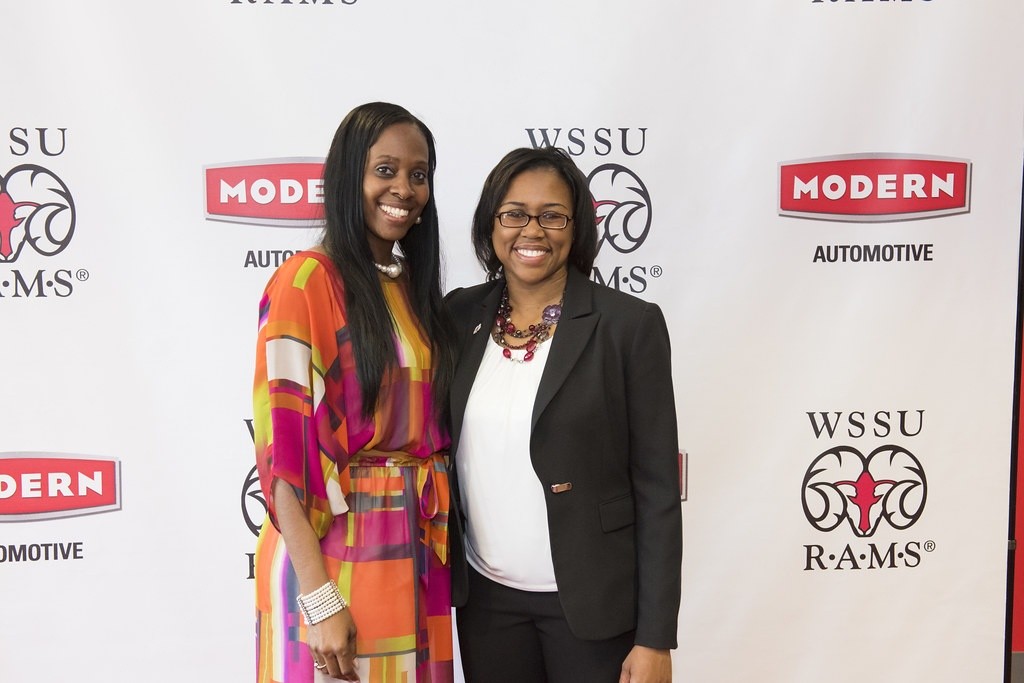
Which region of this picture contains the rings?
[313,660,326,669]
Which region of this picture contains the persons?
[438,142,685,683]
[247,98,456,682]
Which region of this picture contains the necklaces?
[367,252,403,279]
[496,284,564,364]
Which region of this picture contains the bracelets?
[296,577,348,626]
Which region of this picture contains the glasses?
[494,212,574,229]
